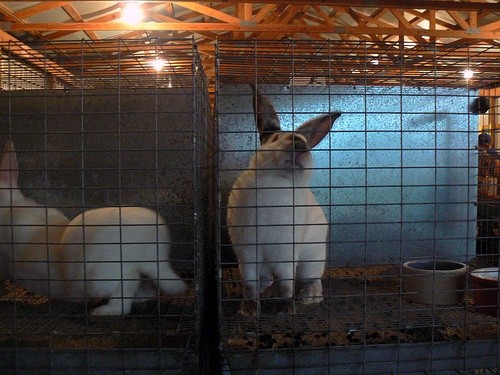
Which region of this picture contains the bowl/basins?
[403,260,467,304]
[470,267,500,316]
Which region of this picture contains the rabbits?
[225,79,344,318]
[0,140,188,319]
